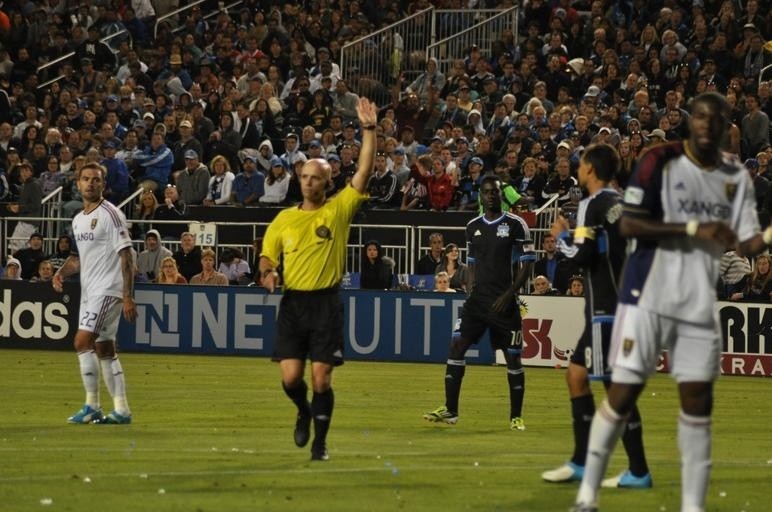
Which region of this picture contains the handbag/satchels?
[514,209,536,228]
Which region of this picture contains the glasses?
[681,63,688,66]
[341,145,353,148]
[167,184,175,187]
[376,153,386,156]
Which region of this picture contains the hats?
[143,98,155,106]
[430,137,446,145]
[238,26,248,32]
[746,159,759,170]
[394,148,406,155]
[596,127,611,136]
[458,86,471,91]
[30,233,43,241]
[121,97,131,103]
[401,124,414,132]
[133,85,144,93]
[96,85,106,94]
[199,59,216,74]
[585,86,600,97]
[80,57,92,63]
[309,139,321,148]
[272,158,282,168]
[456,137,469,144]
[244,155,257,164]
[647,130,668,141]
[104,141,117,149]
[470,157,484,166]
[87,26,99,34]
[246,77,263,84]
[342,123,354,130]
[741,24,756,32]
[107,95,117,103]
[144,113,155,120]
[298,77,310,87]
[190,101,203,110]
[184,150,197,160]
[170,54,182,65]
[326,153,340,162]
[443,120,454,129]
[508,133,520,144]
[319,48,329,55]
[133,119,144,129]
[557,142,571,151]
[178,121,192,128]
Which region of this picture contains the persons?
[259,96,379,461]
[541,141,654,488]
[423,175,538,432]
[570,91,772,511]
[51,162,138,424]
[0,1,771,303]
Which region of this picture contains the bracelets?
[263,269,273,278]
[763,225,772,244]
[361,124,376,130]
[685,219,700,239]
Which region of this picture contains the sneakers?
[511,417,525,431]
[600,470,651,488]
[310,441,328,461]
[294,403,312,447]
[92,412,132,425]
[421,406,457,425]
[542,463,587,482]
[67,404,102,425]
